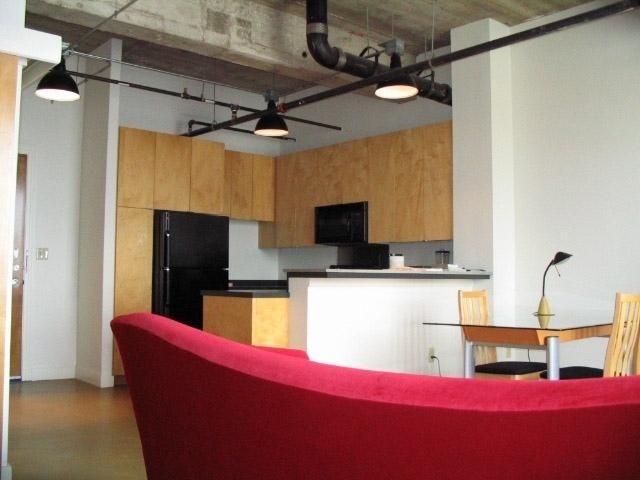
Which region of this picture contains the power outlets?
[429,345,436,364]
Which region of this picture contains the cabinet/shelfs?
[199,287,287,351]
[225,148,276,223]
[114,205,153,378]
[257,127,453,252]
[118,127,225,216]
[281,266,492,378]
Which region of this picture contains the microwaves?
[314,202,367,246]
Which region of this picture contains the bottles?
[433,249,450,269]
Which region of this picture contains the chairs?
[537,291,639,380]
[457,290,546,382]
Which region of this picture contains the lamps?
[533,314,555,329]
[254,99,289,136]
[37,59,80,103]
[533,251,574,315]
[375,54,420,102]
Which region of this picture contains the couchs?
[109,315,640,479]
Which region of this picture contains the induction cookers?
[328,263,388,270]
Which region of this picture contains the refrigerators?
[150,208,231,331]
